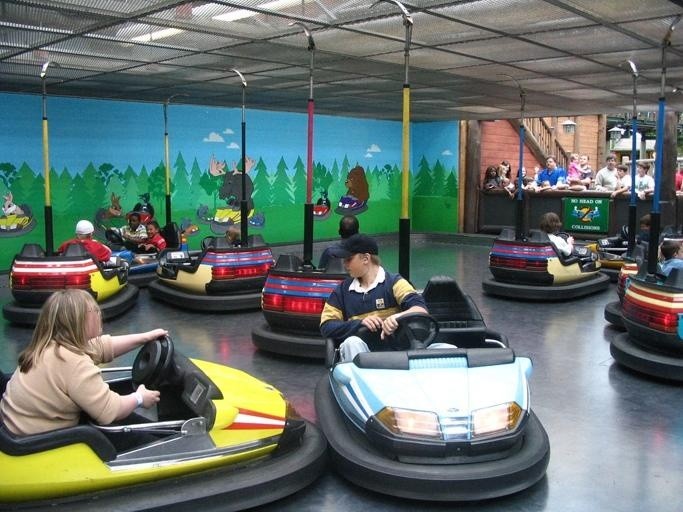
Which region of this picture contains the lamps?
[560,116,575,134]
[606,120,623,145]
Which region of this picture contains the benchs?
[11,240,127,299]
[340,278,501,354]
[617,272,682,357]
[130,221,182,275]
[173,234,274,290]
[492,218,600,275]
[615,241,647,309]
[263,251,354,330]
[0,362,116,468]
[487,231,590,282]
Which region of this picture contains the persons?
[1,288,169,438]
[109,213,147,243]
[482,152,682,201]
[621,214,662,244]
[656,240,682,278]
[538,212,574,257]
[318,234,428,363]
[137,221,166,253]
[58,219,112,263]
[317,214,361,270]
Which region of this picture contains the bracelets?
[134,392,143,407]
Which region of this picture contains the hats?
[332,233,378,258]
[76,220,95,234]
